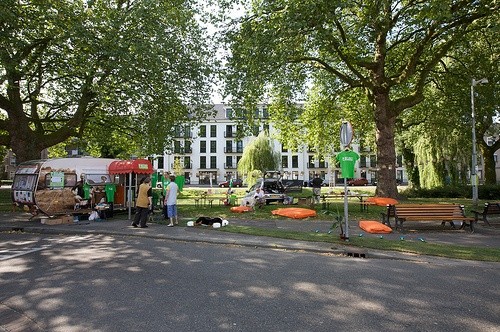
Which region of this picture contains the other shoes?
[167,224,174,227]
[132,223,138,227]
[174,222,178,224]
[141,225,148,228]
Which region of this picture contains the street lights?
[471,77,489,205]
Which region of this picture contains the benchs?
[381,202,500,231]
[29,188,95,222]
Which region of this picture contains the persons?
[254,189,266,209]
[312,174,323,202]
[163,172,179,227]
[71,173,87,199]
[131,176,152,228]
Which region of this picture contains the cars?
[244,170,286,206]
[308,177,329,187]
[373,179,402,186]
[219,179,242,188]
[347,178,368,186]
[157,182,162,188]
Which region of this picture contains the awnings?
[109,159,153,175]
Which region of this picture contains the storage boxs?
[41,216,74,225]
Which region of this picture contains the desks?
[159,192,245,209]
[320,193,398,212]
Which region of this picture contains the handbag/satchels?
[89,211,100,220]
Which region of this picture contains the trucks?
[11,158,153,211]
